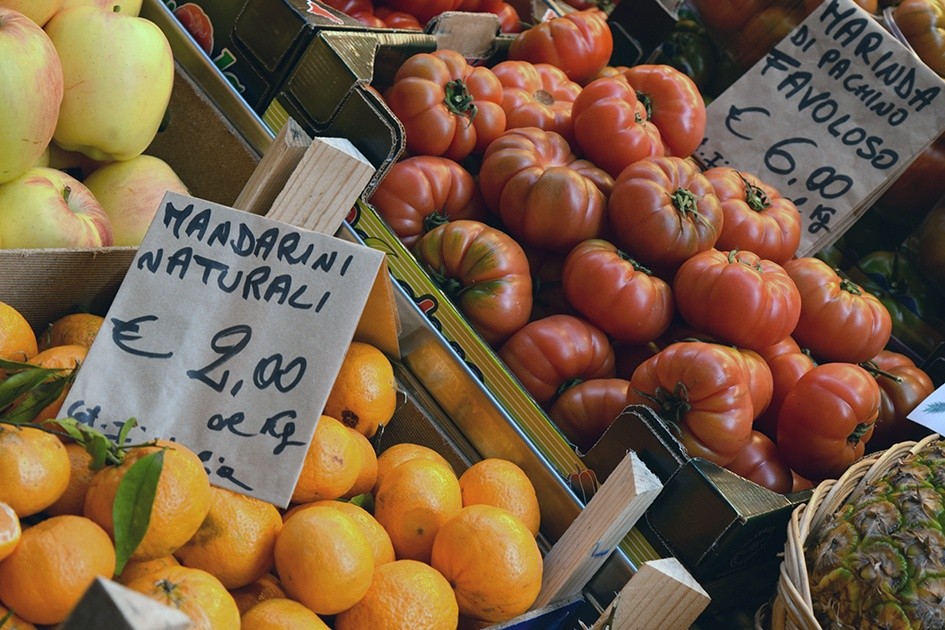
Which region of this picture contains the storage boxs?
[0,0,945,630]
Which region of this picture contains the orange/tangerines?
[1,297,548,628]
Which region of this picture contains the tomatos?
[320,2,932,497]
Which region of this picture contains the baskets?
[758,433,937,629]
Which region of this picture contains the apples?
[1,1,222,245]
